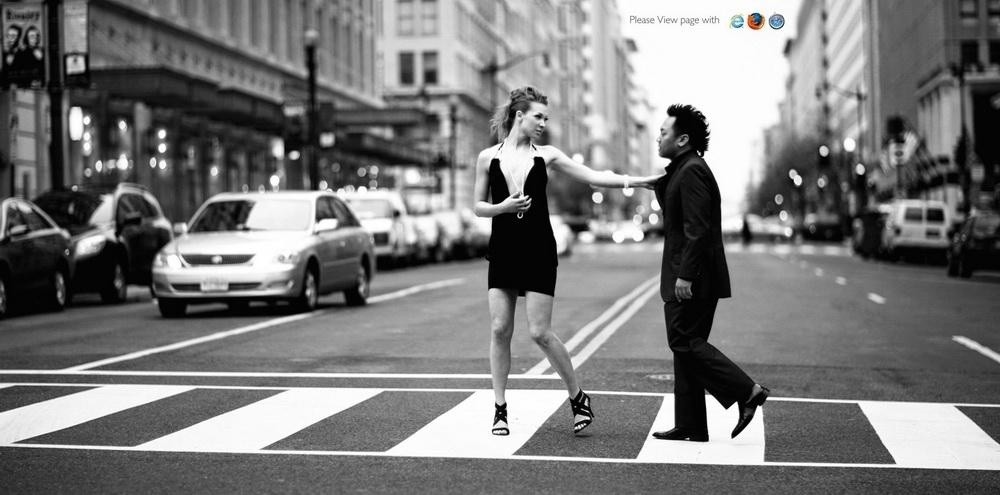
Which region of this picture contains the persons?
[0,24,23,90]
[20,26,46,89]
[652,103,769,443]
[474,85,669,436]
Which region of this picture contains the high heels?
[491,401,510,438]
[568,388,594,435]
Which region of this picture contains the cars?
[721,189,1000,287]
[1,190,82,321]
[149,185,386,315]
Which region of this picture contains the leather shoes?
[729,385,769,443]
[651,426,710,446]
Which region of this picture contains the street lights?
[296,17,333,188]
[471,39,556,148]
[935,46,991,229]
[817,72,872,169]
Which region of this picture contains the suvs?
[24,172,185,309]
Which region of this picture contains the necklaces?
[505,148,528,219]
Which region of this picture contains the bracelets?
[623,175,632,188]
